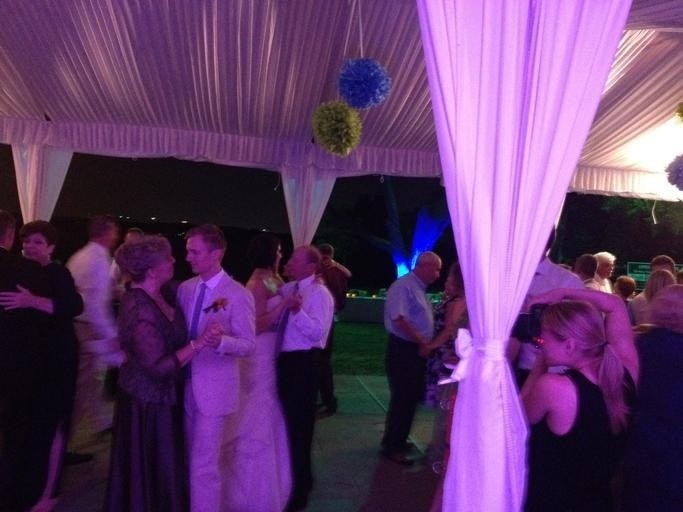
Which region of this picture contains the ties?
[273,282,300,360]
[190,282,207,341]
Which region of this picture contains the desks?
[340,297,385,324]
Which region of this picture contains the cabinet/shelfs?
[627,261,683,291]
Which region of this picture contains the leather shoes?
[379,435,416,453]
[378,444,417,467]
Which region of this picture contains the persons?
[507,225,582,392]
[1,210,353,511]
[378,252,443,466]
[423,265,470,464]
[518,288,641,512]
[577,244,683,512]
